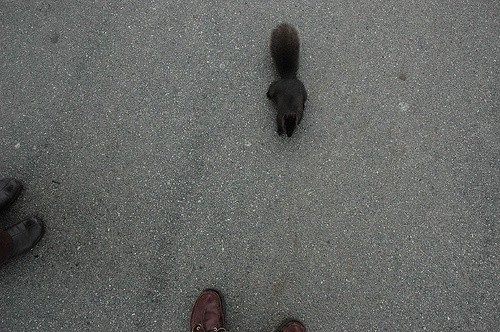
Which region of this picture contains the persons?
[0,177,49,261]
[188,288,306,332]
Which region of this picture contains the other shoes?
[191,288,226,332]
[0,216,44,266]
[274,317,307,332]
[0,177,22,214]
